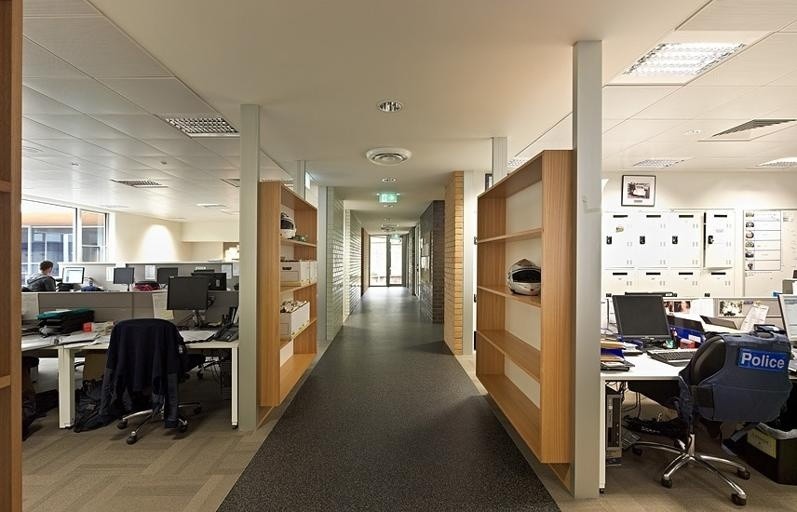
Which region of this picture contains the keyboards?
[654,351,696,361]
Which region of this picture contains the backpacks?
[74,377,125,433]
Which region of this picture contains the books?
[601,358,635,371]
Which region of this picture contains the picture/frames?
[621,175,657,207]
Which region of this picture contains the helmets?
[506,259,541,297]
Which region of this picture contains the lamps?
[366,148,411,166]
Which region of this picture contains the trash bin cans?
[746,422,797,485]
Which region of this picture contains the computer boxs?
[220,358,232,400]
[606,385,623,468]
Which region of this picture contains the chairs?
[622,335,791,506]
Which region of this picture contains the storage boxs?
[309,261,318,284]
[281,260,309,288]
[737,421,797,485]
[280,301,310,340]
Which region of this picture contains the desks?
[599,296,797,492]
[21,263,238,443]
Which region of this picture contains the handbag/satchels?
[620,414,673,437]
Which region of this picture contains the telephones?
[212,326,238,342]
[757,325,786,335]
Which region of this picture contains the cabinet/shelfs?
[475,150,574,466]
[257,180,318,407]
[602,208,746,334]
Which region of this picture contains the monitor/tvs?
[60,266,227,330]
[610,322,611,324]
[610,290,797,355]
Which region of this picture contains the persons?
[677,301,690,312]
[25,261,57,291]
[665,301,672,312]
